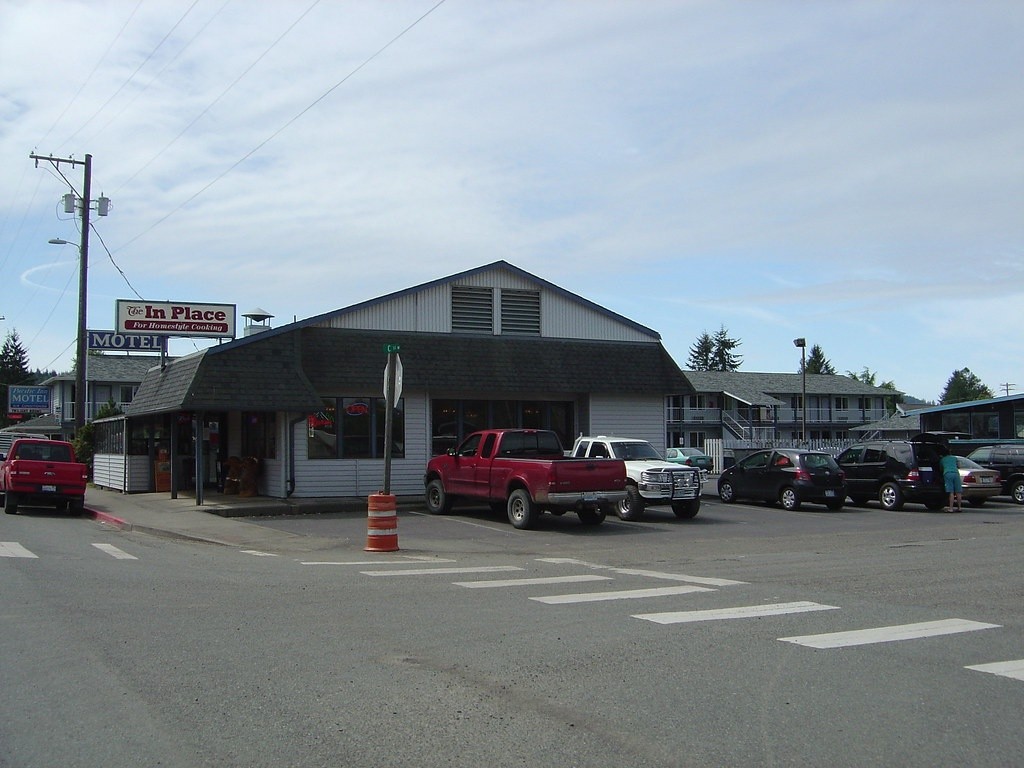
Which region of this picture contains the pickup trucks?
[562,431,710,522]
[422,428,629,532]
[0,437,88,517]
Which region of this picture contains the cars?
[717,447,848,512]
[951,455,1004,506]
[666,446,714,473]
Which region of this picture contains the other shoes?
[944,508,953,513]
[955,508,962,512]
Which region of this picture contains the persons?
[937,449,962,513]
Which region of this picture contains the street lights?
[48,238,85,438]
[794,338,807,445]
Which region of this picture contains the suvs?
[835,430,971,512]
[962,443,1024,505]
